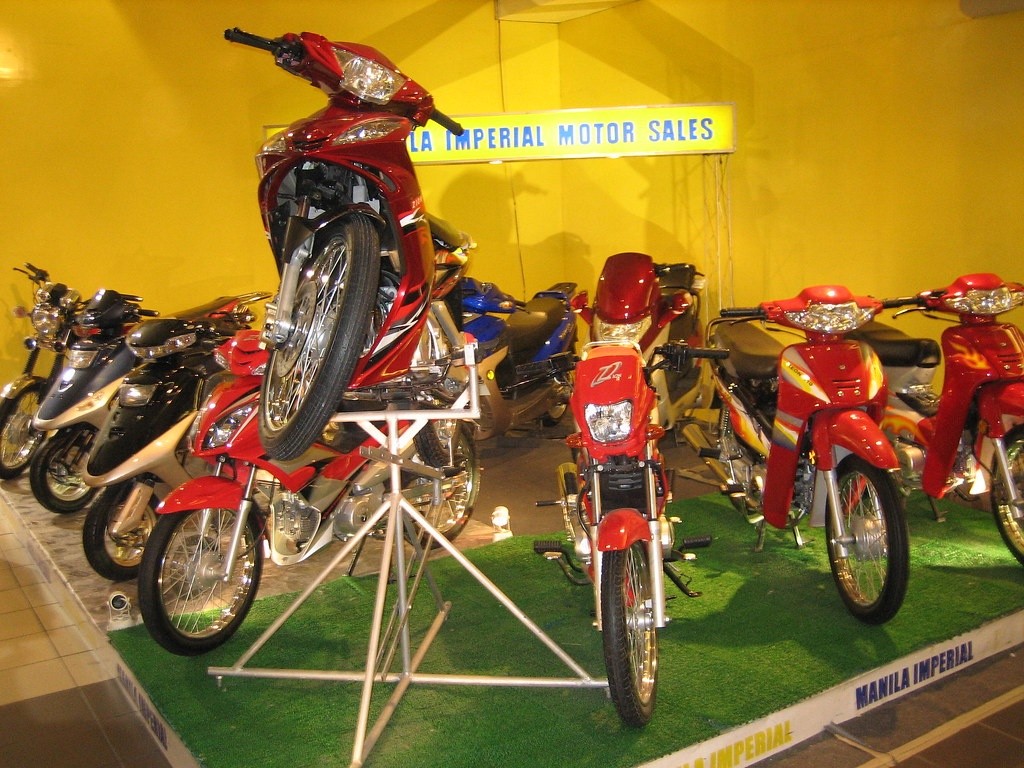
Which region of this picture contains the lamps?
[491,506,514,541]
[106,592,134,630]
